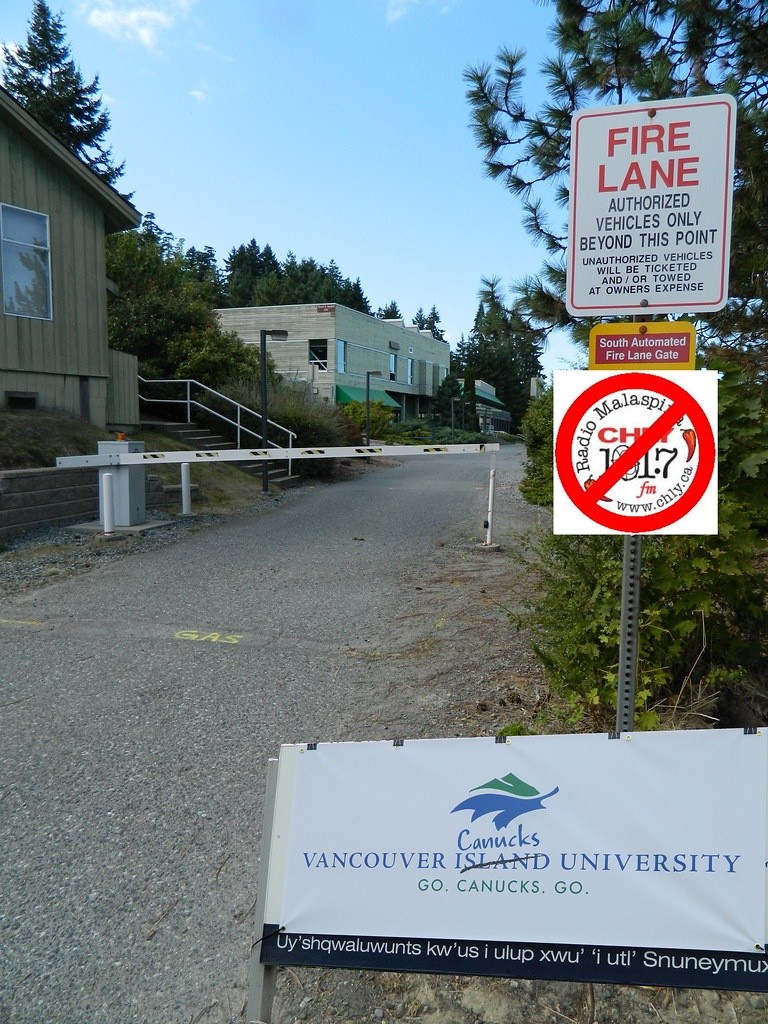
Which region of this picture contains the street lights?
[485,406,491,432]
[366,371,383,463]
[452,397,460,439]
[259,330,289,489]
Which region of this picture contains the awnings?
[476,388,504,405]
[336,384,402,407]
